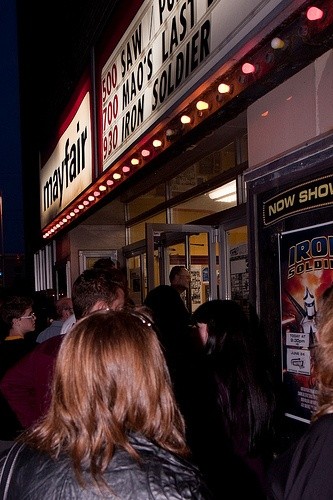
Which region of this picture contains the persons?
[0,311,206,500]
[0,294,37,442]
[277,284,333,500]
[178,299,301,500]
[36,298,75,344]
[95,257,115,270]
[0,270,124,429]
[169,266,191,305]
[143,285,224,500]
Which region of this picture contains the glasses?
[20,313,35,321]
[89,308,154,326]
